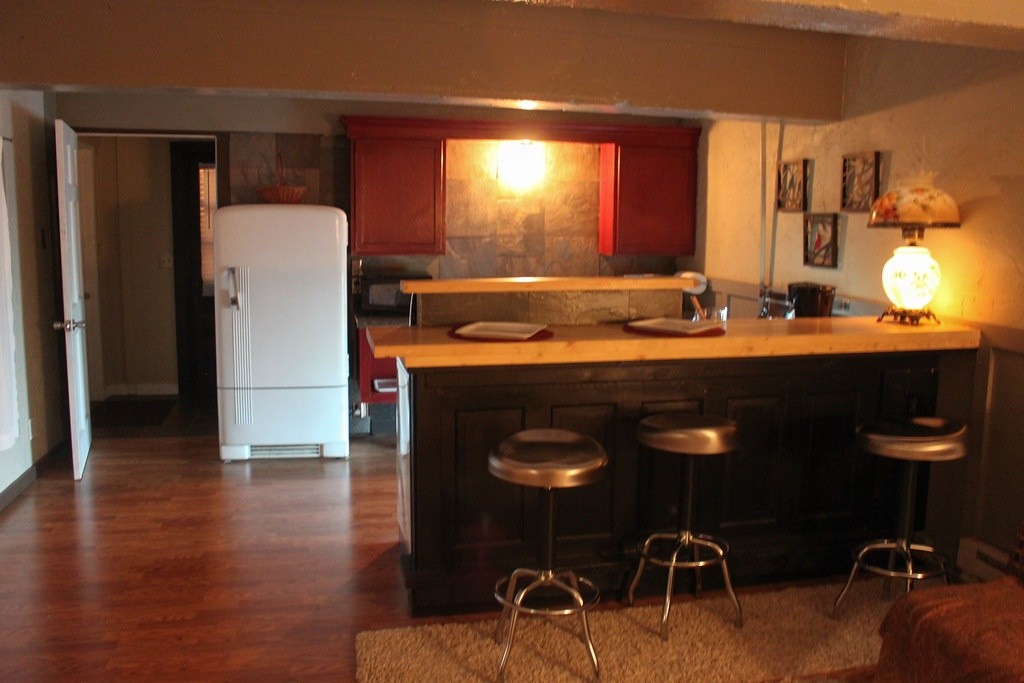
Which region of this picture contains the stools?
[834,410,968,621]
[624,412,750,635]
[491,429,610,682]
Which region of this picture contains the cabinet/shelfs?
[343,117,447,254]
[598,122,699,258]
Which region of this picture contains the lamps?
[865,180,961,326]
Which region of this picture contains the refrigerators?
[212,193,350,461]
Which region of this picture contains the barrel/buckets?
[790,278,836,318]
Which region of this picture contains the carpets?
[354,563,944,683]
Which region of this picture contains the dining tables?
[366,314,978,624]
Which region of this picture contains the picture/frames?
[803,212,841,268]
[776,157,807,213]
[839,150,879,214]
